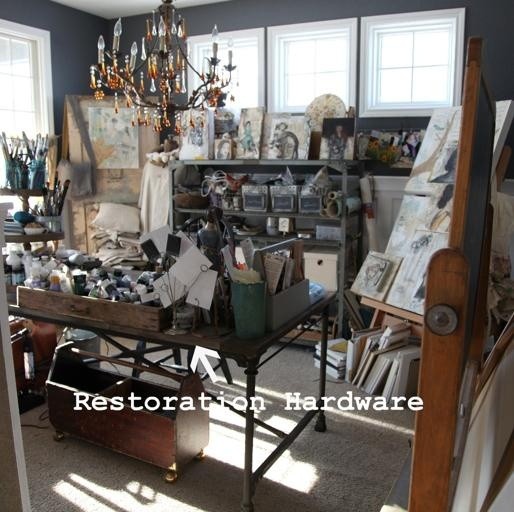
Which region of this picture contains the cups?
[6,160,46,193]
[24,226,50,236]
[37,216,63,233]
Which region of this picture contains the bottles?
[72,274,88,296]
[4,247,62,292]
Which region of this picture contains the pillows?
[91,202,141,236]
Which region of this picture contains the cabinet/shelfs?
[0,138,67,287]
[165,158,366,352]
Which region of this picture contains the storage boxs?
[16,266,175,329]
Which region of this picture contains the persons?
[195,205,227,251]
[361,260,380,285]
[216,120,350,160]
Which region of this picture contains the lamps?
[87,0,236,136]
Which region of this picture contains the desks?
[5,248,339,511]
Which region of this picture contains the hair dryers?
[58,250,169,305]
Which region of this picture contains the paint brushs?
[0,132,48,189]
[20,179,70,216]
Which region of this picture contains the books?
[313,320,420,406]
